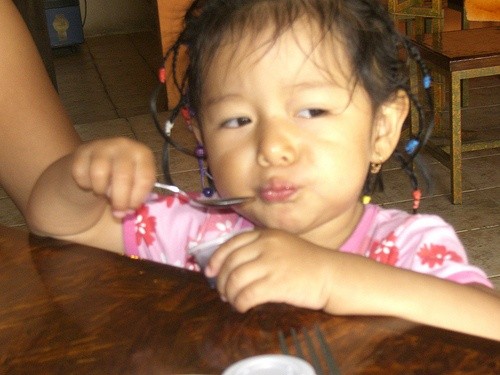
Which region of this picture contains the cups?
[223,354,316,374]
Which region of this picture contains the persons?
[26,0,499,342]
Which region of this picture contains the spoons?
[154,182,256,210]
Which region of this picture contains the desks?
[1,226,500,375]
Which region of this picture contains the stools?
[407,26,500,207]
[374,1,447,116]
[460,0,499,108]
